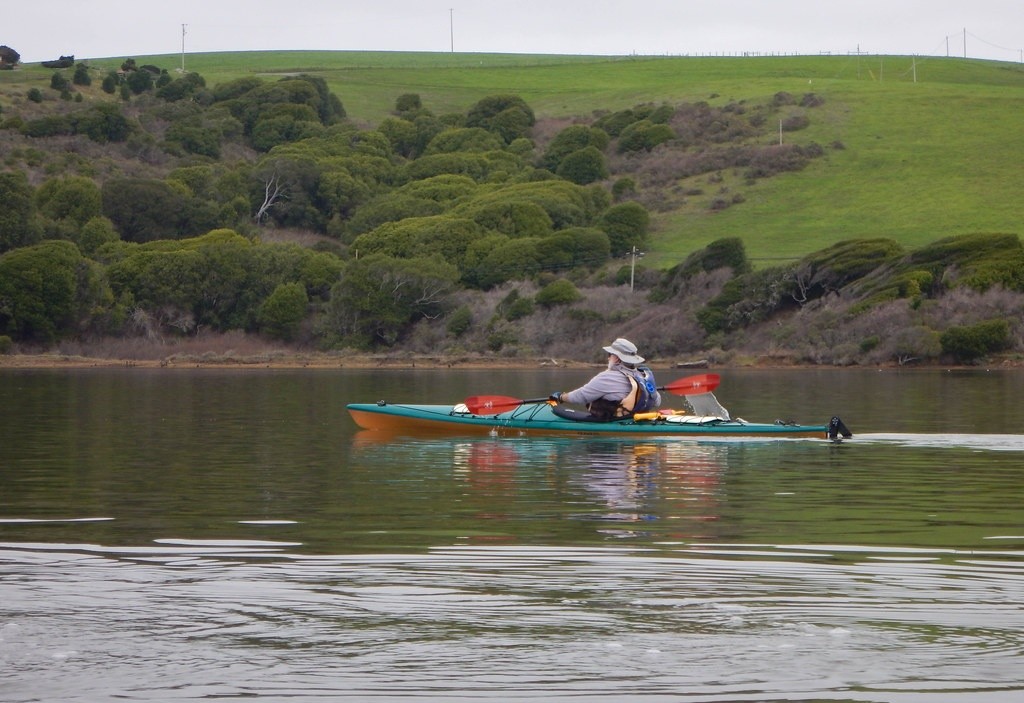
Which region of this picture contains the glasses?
[608,353,614,357]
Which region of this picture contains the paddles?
[464,372,721,418]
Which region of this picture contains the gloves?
[549,392,562,401]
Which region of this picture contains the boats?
[345,399,854,445]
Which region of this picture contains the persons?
[548,338,661,418]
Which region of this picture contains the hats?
[603,338,645,364]
